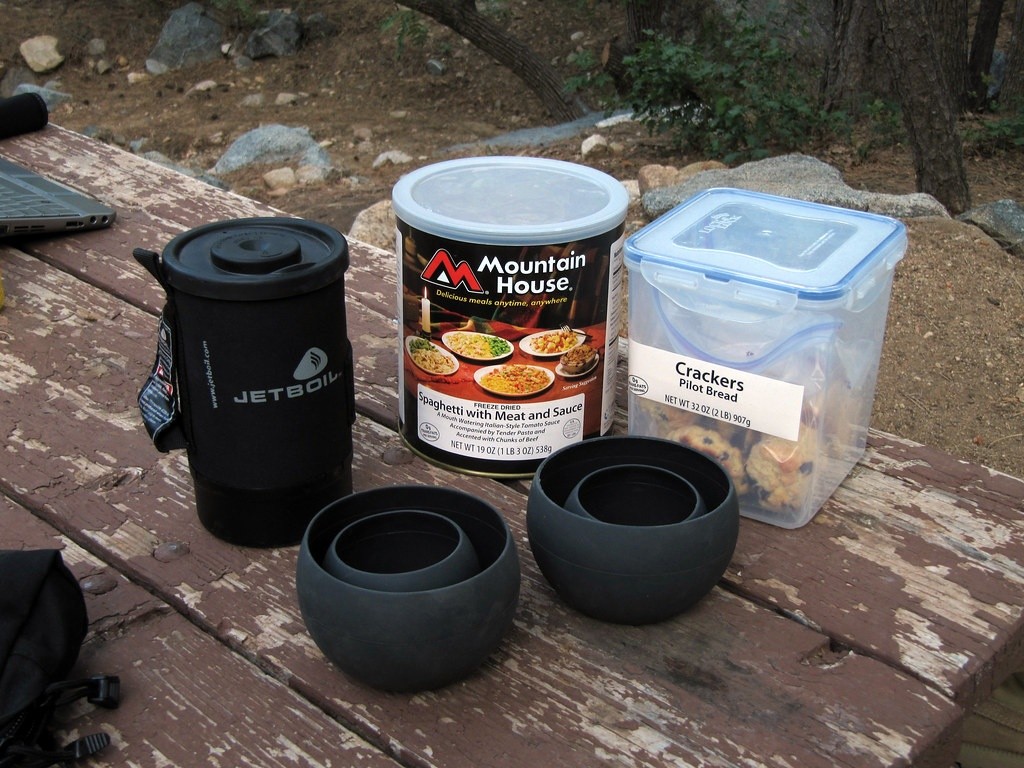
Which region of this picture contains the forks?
[558,324,593,338]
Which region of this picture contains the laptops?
[0,156,116,238]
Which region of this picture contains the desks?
[0,114,1024,768]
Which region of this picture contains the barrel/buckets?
[165,218,359,549]
[390,160,633,478]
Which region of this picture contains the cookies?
[638,371,859,511]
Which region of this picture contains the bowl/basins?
[525,434,741,624]
[563,463,706,527]
[296,484,522,696]
[321,509,481,593]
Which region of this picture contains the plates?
[473,364,556,396]
[518,328,587,356]
[441,331,514,361]
[554,351,600,378]
[404,335,459,375]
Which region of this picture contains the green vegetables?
[484,336,511,356]
[409,338,439,353]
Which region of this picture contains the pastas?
[411,348,456,373]
